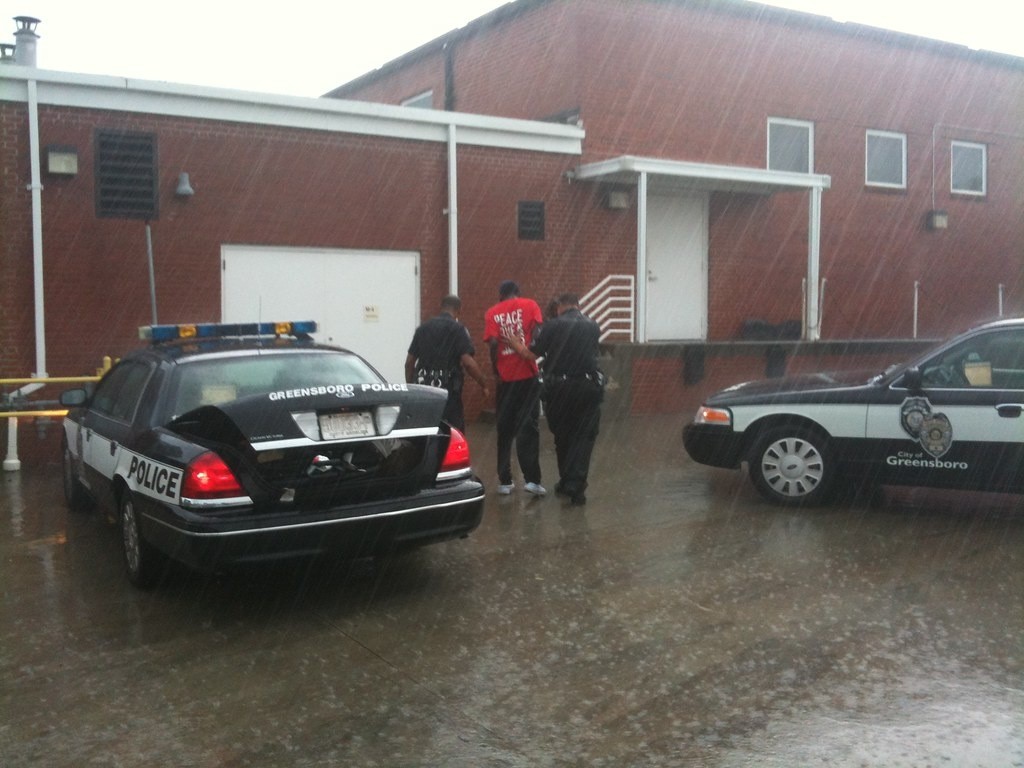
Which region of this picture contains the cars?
[60,318,485,594]
[682,317,1024,505]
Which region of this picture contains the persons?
[405,295,489,436]
[484,281,547,495]
[500,293,602,505]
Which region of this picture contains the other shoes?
[554,482,568,492]
[573,494,586,507]
[524,482,547,495]
[497,480,515,493]
